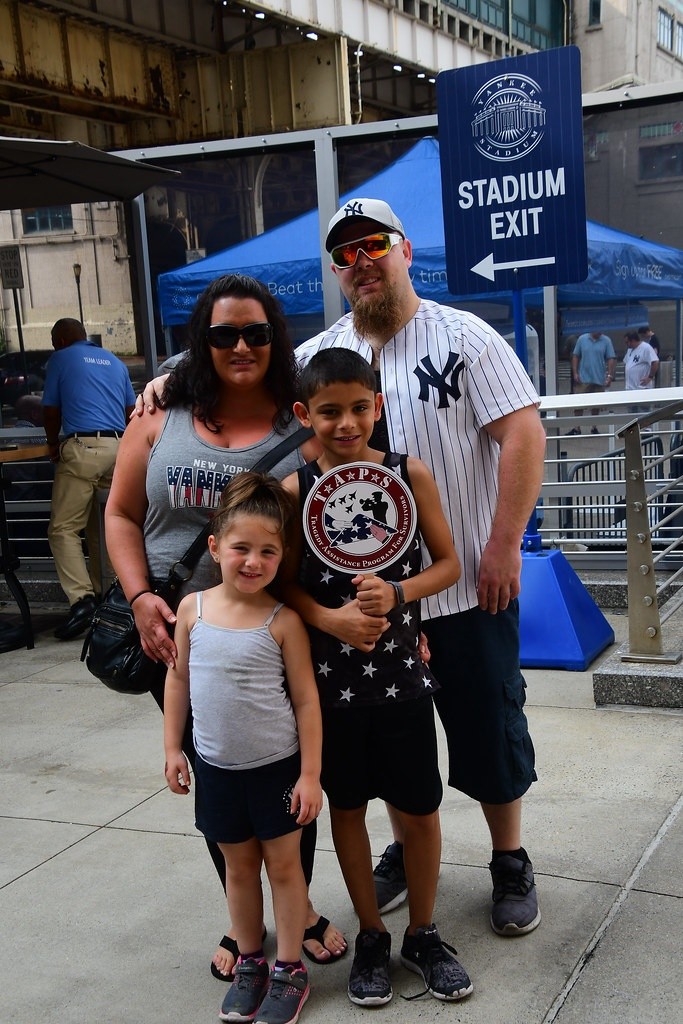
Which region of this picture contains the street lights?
[72,264,84,325]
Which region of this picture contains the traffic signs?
[434,44,590,295]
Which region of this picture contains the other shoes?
[590,427,600,434]
[54,597,95,641]
[641,428,653,439]
[565,429,581,436]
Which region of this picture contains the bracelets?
[608,375,613,380]
[387,581,405,608]
[649,375,653,378]
[129,589,152,607]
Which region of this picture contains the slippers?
[302,916,347,965]
[210,927,268,982]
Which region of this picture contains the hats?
[325,199,405,252]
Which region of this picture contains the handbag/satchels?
[80,570,185,695]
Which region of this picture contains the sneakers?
[397,923,474,1000]
[489,854,541,935]
[252,963,310,1024]
[218,955,270,1022]
[347,928,392,1005]
[367,839,442,915]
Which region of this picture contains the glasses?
[205,323,274,349]
[330,232,402,268]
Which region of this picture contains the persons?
[638,326,660,360]
[266,347,474,1006]
[562,328,616,435]
[41,317,137,638]
[165,470,323,1024]
[622,330,659,434]
[132,196,547,935]
[102,275,431,979]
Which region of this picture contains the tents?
[156,131,683,386]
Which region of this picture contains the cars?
[2,349,59,404]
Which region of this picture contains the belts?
[66,431,124,439]
